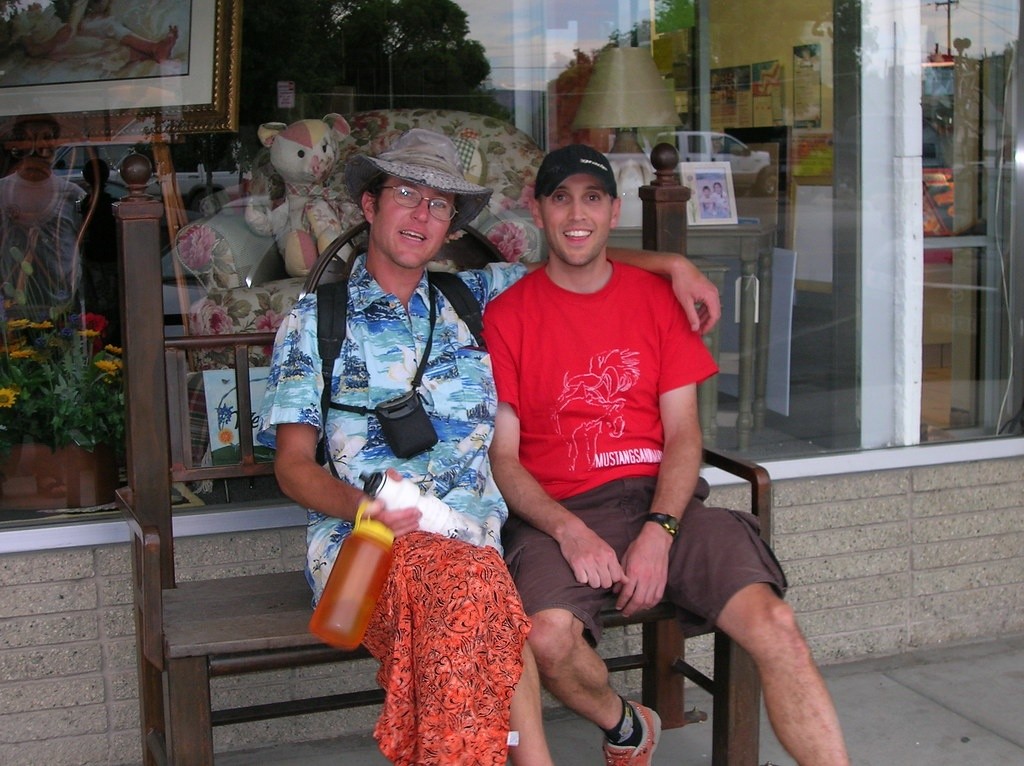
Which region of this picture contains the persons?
[484,144,856,766]
[10,1,179,70]
[255,126,722,766]
[1,114,89,340]
[698,182,729,217]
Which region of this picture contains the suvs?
[652,132,779,195]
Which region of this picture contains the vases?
[0,443,120,509]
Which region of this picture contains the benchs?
[97,143,775,765]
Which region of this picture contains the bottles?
[359,472,485,545]
[308,501,394,650]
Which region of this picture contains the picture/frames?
[0,0,241,151]
[678,160,740,226]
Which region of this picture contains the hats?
[346,128,494,233]
[535,144,617,203]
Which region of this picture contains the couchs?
[177,107,546,371]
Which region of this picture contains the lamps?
[570,44,679,231]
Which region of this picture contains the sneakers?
[602,701,662,766]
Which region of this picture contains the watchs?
[645,512,680,538]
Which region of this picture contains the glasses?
[380,185,459,221]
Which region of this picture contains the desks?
[608,225,780,441]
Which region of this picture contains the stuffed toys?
[242,111,352,274]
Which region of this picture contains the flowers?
[0,299,124,453]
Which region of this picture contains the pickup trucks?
[53,135,241,220]
